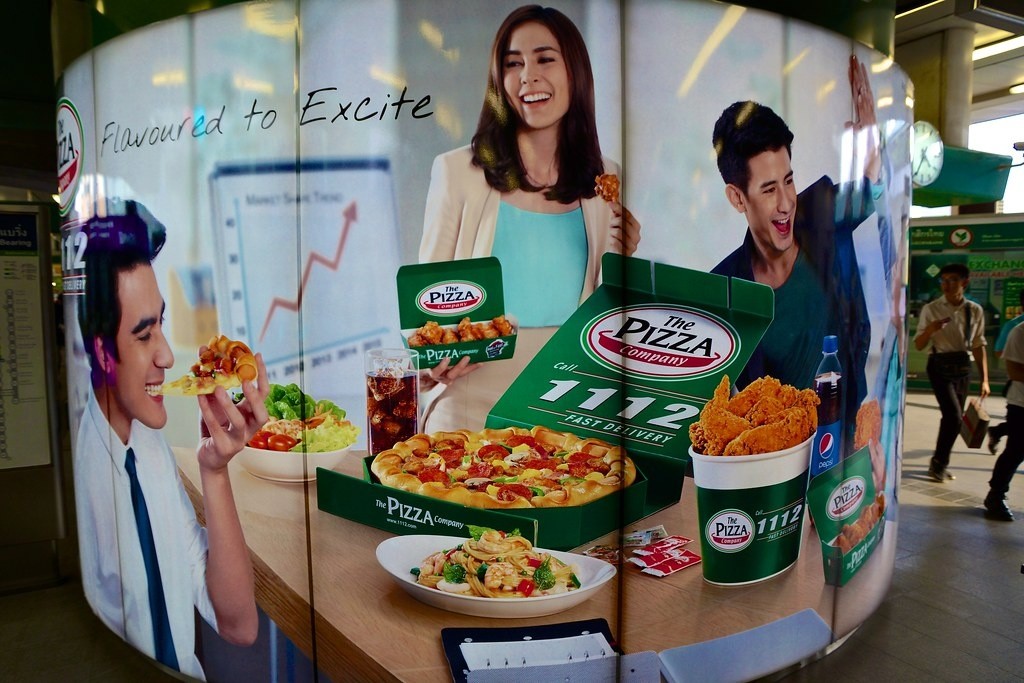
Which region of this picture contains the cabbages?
[286,416,360,452]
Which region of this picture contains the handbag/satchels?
[926,302,972,379]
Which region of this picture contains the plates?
[374,534,617,618]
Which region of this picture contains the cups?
[365,348,421,456]
[688,433,816,586]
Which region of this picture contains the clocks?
[912,120,944,187]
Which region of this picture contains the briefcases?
[961,395,991,449]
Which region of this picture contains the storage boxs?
[317,251,775,558]
[396,256,519,371]
[807,445,888,588]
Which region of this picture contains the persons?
[709,54,884,518]
[983,320,1024,523]
[911,265,991,484]
[72,202,267,683]
[988,286,1024,454]
[55,292,66,369]
[862,65,900,527]
[412,4,646,440]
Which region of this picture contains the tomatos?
[246,430,296,452]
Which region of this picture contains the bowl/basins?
[235,443,352,481]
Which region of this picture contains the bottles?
[808,336,842,529]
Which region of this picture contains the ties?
[124,447,180,671]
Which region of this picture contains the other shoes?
[984,492,1013,519]
[927,469,956,483]
[988,426,999,454]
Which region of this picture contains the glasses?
[937,277,963,284]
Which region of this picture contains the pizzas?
[154,335,258,396]
[370,426,636,510]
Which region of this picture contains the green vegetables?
[232,384,347,421]
[442,525,556,591]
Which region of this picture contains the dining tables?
[167,324,899,683]
